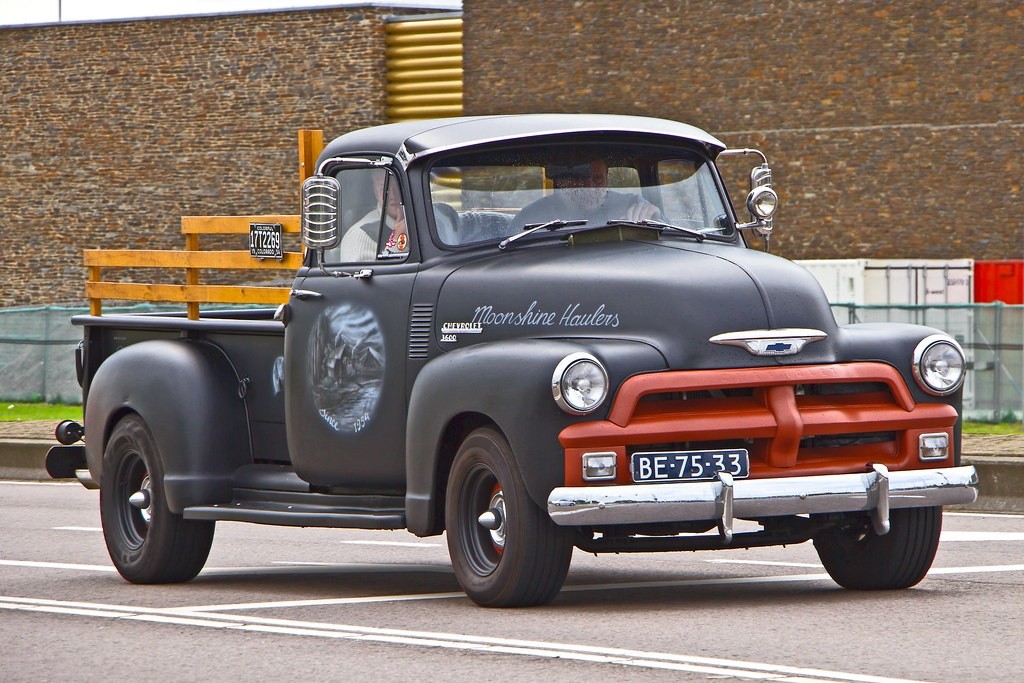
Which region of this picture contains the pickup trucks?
[43,112,978,613]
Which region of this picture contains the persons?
[508,155,700,234]
[341,169,455,263]
